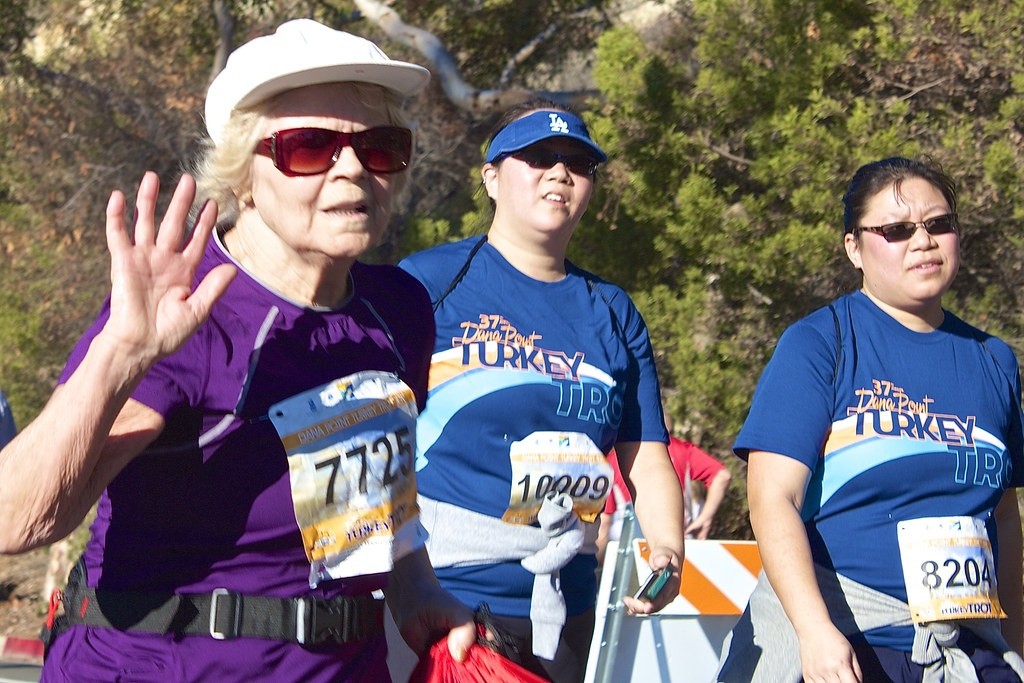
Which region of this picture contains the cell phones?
[634,566,673,602]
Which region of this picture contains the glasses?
[852,212,960,243]
[249,126,413,176]
[509,145,599,179]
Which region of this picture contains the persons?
[0,19,732,683]
[709,154,1024,683]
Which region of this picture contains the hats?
[204,18,430,147]
[485,108,608,164]
